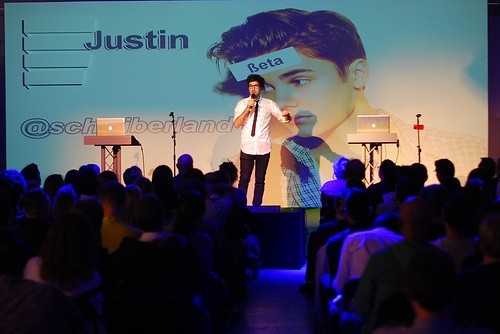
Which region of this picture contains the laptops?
[96,118,125,135]
[357,115,390,134]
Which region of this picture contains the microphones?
[169,112,174,117]
[251,93,255,99]
[416,114,421,117]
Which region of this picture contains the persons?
[233,74,292,206]
[316,158,500,334]
[207,8,489,207]
[298,157,351,299]
[0,154,261,334]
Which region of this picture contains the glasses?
[249,84,261,89]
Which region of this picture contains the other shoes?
[299,284,316,295]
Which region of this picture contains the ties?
[251,100,259,137]
